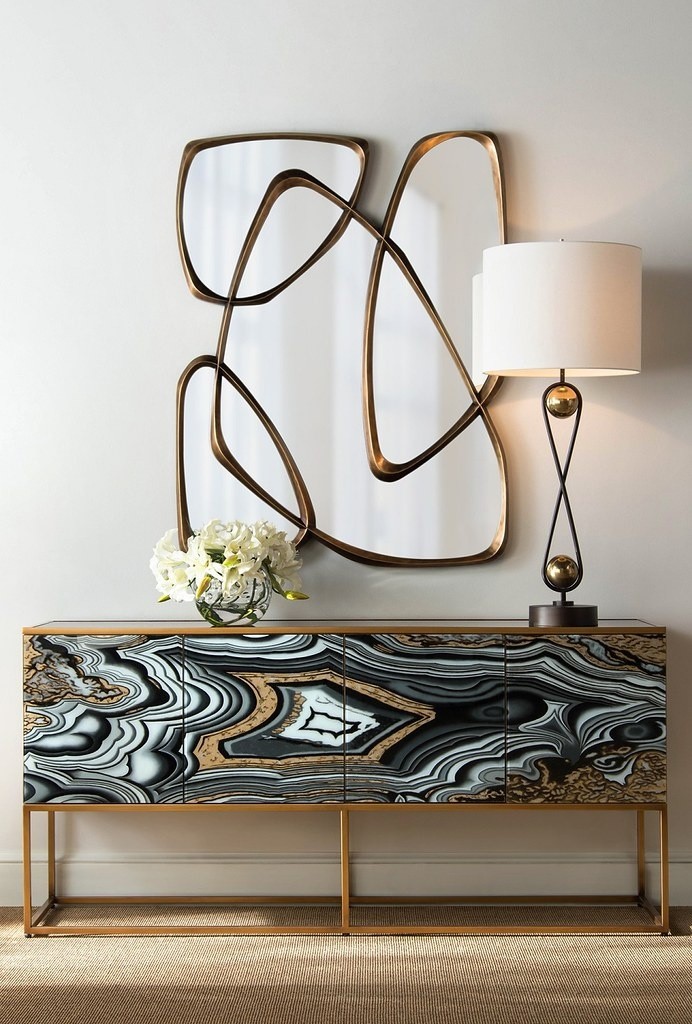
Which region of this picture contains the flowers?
[148,519,310,626]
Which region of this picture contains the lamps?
[481,239,643,629]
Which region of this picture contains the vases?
[191,564,273,626]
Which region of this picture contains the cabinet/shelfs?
[22,619,671,935]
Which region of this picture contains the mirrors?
[174,130,510,569]
